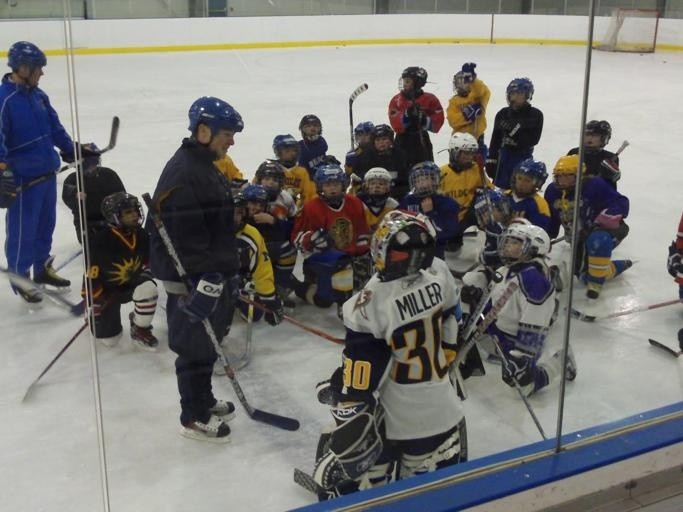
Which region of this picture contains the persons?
[664,214,683,354]
[63,63,643,503]
[0,41,101,309]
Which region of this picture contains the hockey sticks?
[349,83,368,149]
[648,337,682,357]
[216,292,254,378]
[239,294,346,346]
[21,115,122,188]
[21,319,88,400]
[142,194,300,432]
[564,298,682,323]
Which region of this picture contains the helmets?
[312,164,348,203]
[452,70,477,97]
[73,142,102,178]
[496,221,553,268]
[187,95,245,136]
[299,114,322,142]
[6,40,47,67]
[512,153,587,198]
[273,134,301,168]
[255,159,288,201]
[582,119,612,155]
[354,122,394,157]
[448,131,479,174]
[362,166,393,201]
[230,184,269,231]
[367,208,439,282]
[407,162,442,199]
[504,77,535,111]
[398,66,428,93]
[99,191,145,231]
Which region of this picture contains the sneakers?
[5,272,44,303]
[177,412,232,444]
[30,255,71,289]
[562,343,577,382]
[586,287,599,299]
[127,312,161,352]
[207,400,235,421]
